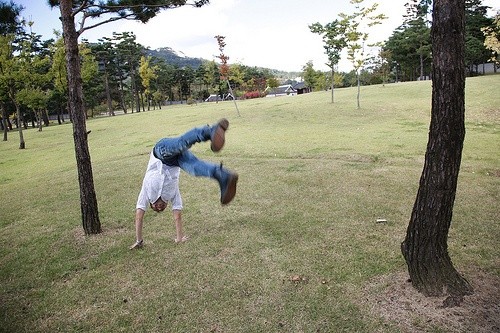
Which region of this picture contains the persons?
[129,118,240,252]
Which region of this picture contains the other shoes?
[210,119,228,151]
[218,170,239,205]
